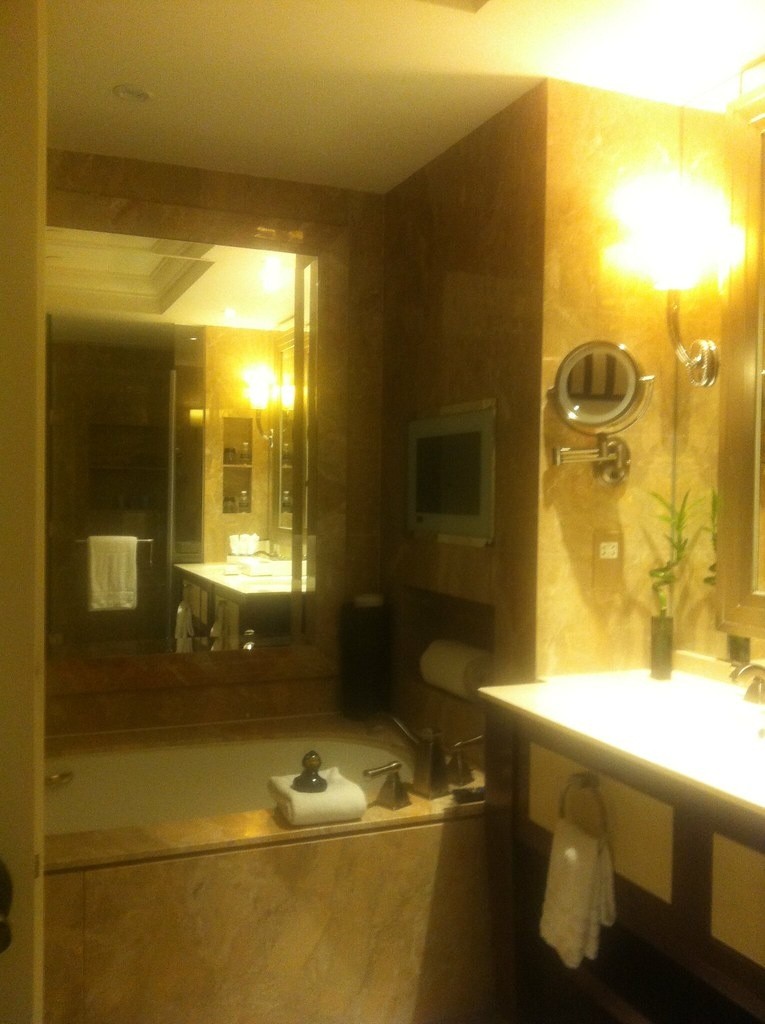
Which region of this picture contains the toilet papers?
[413,638,492,701]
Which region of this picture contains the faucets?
[365,710,451,802]
[729,659,763,698]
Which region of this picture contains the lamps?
[600,162,744,388]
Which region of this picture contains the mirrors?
[672,295,765,669]
[45,224,316,661]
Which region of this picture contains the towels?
[539,817,619,969]
[173,602,195,640]
[87,535,138,612]
[266,766,368,828]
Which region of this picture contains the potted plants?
[702,489,751,664]
[638,485,706,680]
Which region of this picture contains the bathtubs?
[46,716,484,1023]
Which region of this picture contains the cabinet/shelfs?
[202,404,271,561]
[482,707,765,1024]
[178,581,307,653]
